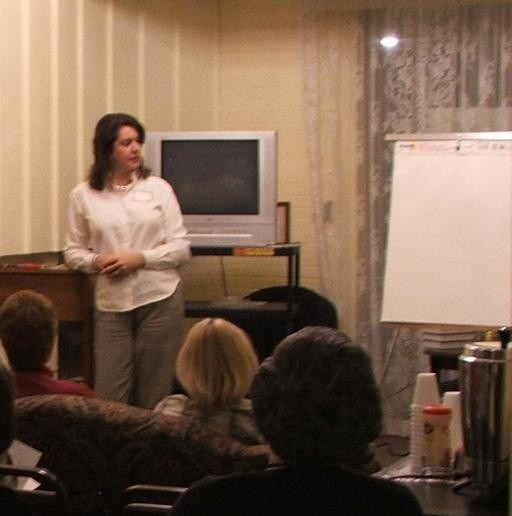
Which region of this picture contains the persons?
[149,316,268,442]
[61,112,192,410]
[1,286,96,399]
[165,325,425,515]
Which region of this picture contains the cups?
[410,372,463,475]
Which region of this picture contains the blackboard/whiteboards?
[378,131,512,329]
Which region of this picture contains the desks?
[3,266,93,387]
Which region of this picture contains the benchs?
[0,393,271,468]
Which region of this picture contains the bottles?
[423,403,452,470]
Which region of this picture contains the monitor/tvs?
[143,130,277,249]
